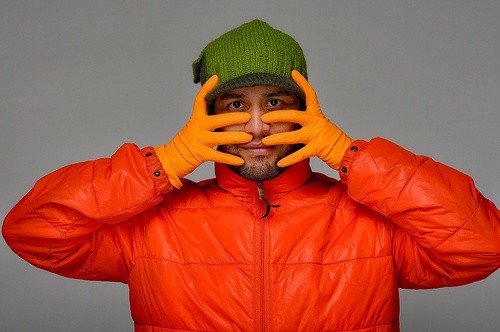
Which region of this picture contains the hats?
[192,20,308,98]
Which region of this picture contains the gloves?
[154,75,252,189]
[261,70,353,170]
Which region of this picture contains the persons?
[0,19,500,332]
